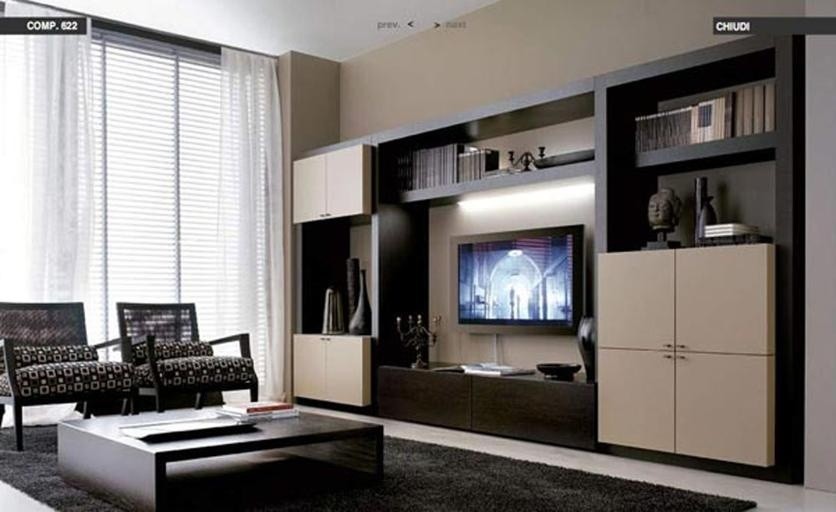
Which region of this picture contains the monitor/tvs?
[447,223,587,335]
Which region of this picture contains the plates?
[536,364,582,381]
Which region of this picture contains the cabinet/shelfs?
[596,244,779,471]
[376,366,598,457]
[594,34,800,249]
[287,333,373,414]
[290,143,375,229]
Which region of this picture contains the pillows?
[1,346,98,373]
[131,342,214,368]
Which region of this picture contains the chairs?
[1,302,138,451]
[116,301,259,413]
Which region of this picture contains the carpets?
[0,411,760,511]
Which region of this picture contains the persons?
[648,188,682,231]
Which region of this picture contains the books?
[119,415,259,441]
[633,82,775,152]
[701,221,773,247]
[218,403,298,421]
[432,362,536,376]
[399,144,508,191]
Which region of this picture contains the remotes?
[432,367,463,373]
